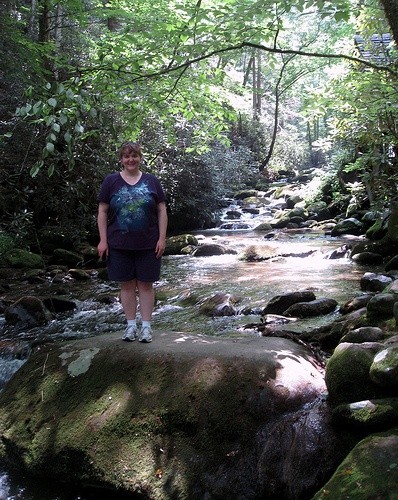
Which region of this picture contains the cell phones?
[102,250,107,262]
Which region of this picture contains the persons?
[98,142,168,344]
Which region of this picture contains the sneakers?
[138,326,153,343]
[122,325,138,341]
